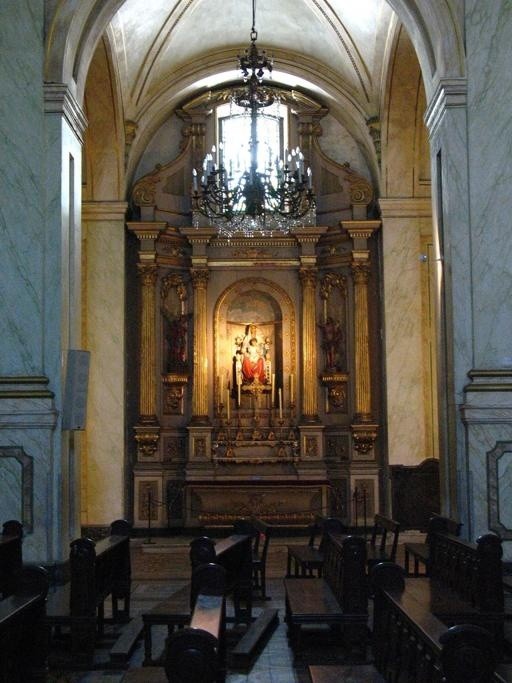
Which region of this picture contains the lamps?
[191,0,316,240]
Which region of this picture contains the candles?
[291,374,294,403]
[279,388,283,418]
[272,374,275,402]
[227,390,230,420]
[220,376,223,403]
[238,375,241,405]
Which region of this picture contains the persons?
[244,338,268,385]
